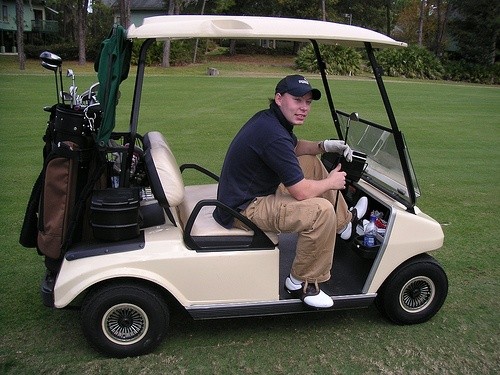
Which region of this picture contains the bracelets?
[318,140,325,151]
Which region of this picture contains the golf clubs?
[40,51,100,110]
[334,111,360,213]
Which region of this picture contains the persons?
[217,75,368,307]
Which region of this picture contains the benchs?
[142,129,280,251]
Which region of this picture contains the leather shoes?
[339,197,368,241]
[284,273,334,309]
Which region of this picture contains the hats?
[275,75,321,101]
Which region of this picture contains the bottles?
[363,214,377,246]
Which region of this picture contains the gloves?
[324,139,353,162]
